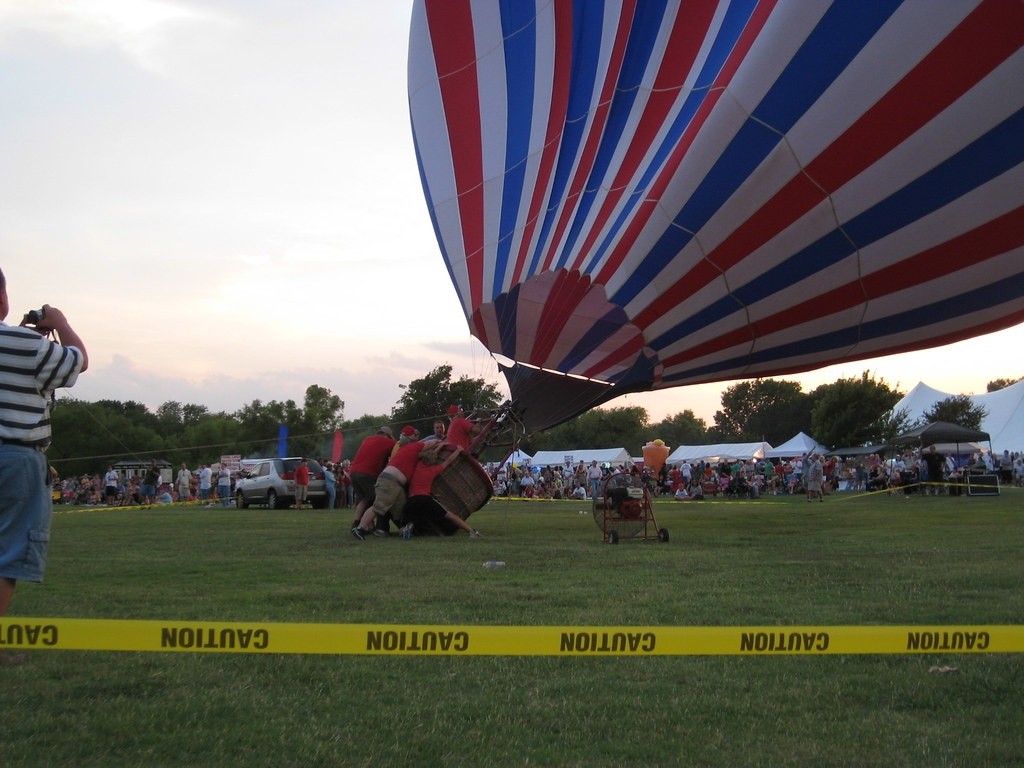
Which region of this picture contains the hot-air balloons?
[398,0,1024,536]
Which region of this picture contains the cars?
[234,457,330,509]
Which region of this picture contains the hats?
[380,426,394,439]
[448,404,462,416]
[402,425,415,436]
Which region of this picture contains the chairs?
[1014,468,1024,487]
[703,477,749,501]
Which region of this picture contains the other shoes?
[469,529,481,537]
[352,527,364,540]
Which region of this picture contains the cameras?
[28,309,46,324]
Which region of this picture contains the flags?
[277,424,288,458]
[331,431,344,463]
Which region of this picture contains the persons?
[0,269,89,619]
[349,421,484,541]
[477,452,1024,498]
[445,403,483,456]
[45,455,361,510]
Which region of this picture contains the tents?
[823,442,899,458]
[531,447,634,472]
[505,447,533,480]
[631,456,645,463]
[921,441,981,456]
[665,441,774,467]
[487,461,509,481]
[859,376,1024,455]
[765,431,831,458]
[889,420,999,497]
